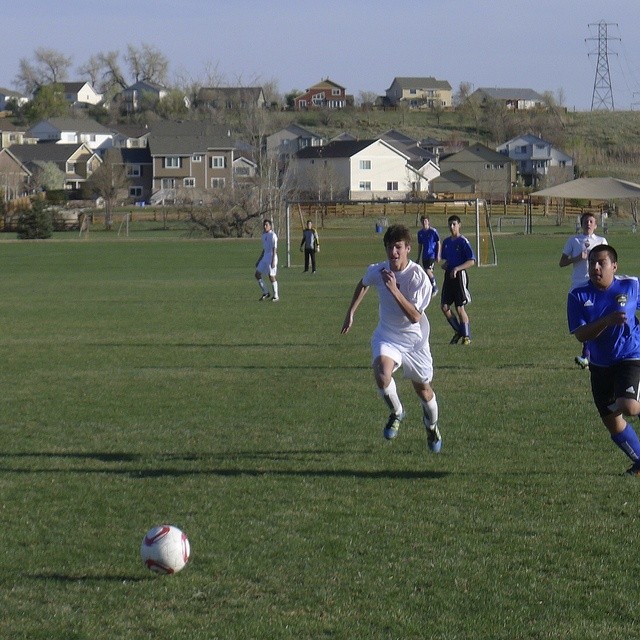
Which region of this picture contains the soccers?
[140,524,190,575]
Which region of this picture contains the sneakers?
[623,463,640,477]
[462,336,471,346]
[423,416,442,454]
[271,297,280,302]
[574,355,588,368]
[384,410,404,439]
[450,331,461,344]
[432,289,439,297]
[259,292,270,301]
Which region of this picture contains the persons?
[575,214,582,234]
[559,213,609,370]
[440,215,476,345]
[300,220,320,274]
[601,211,609,235]
[567,244,639,475]
[255,219,279,302]
[340,224,442,453]
[415,216,440,296]
